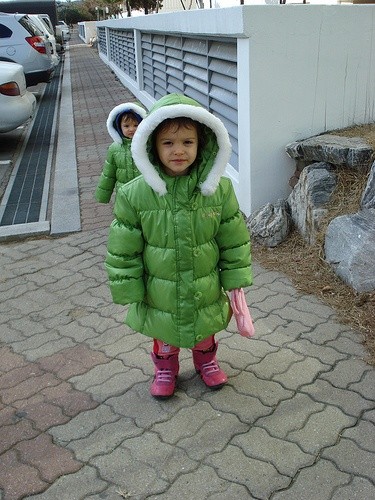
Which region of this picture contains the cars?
[0,13,71,89]
[0,61,37,133]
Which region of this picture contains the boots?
[193,342,227,389]
[151,351,179,399]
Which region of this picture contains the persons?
[104,93,253,399]
[95,100,148,204]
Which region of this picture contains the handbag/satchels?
[231,288,255,337]
